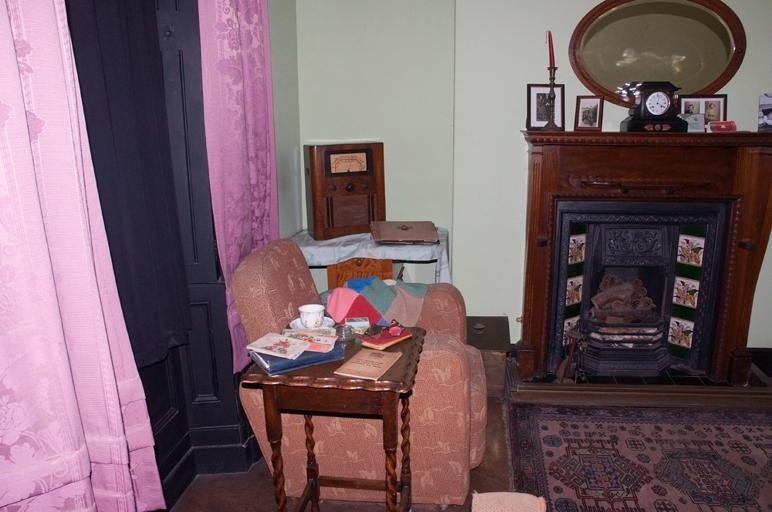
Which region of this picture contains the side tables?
[240,327,426,512]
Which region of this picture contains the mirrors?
[567,0,747,109]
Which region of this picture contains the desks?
[288,228,453,284]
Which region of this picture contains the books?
[369,220,441,246]
[245,316,414,382]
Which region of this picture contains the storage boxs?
[481,350,508,403]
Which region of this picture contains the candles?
[545,30,555,67]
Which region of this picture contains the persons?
[706,102,717,124]
[688,103,695,113]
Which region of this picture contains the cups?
[298,303,326,329]
[334,324,355,342]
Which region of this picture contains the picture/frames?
[574,96,604,134]
[526,83,565,131]
[678,94,728,133]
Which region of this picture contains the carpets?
[508,405,772,512]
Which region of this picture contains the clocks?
[620,81,688,133]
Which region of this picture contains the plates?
[292,317,334,330]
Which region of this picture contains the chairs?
[327,257,392,290]
[230,240,490,512]
